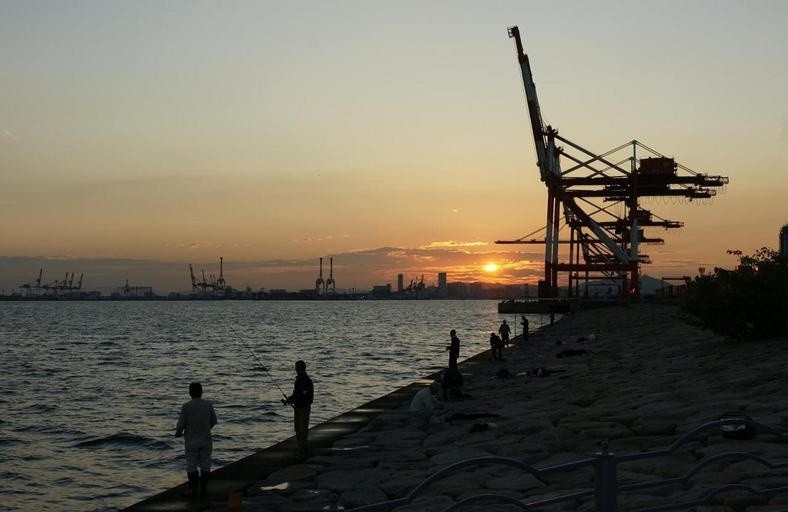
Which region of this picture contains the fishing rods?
[190,281,295,412]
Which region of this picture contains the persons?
[519,315,529,340]
[284,359,313,447]
[489,333,503,361]
[445,330,459,369]
[498,320,512,349]
[174,383,217,497]
[407,381,449,430]
[433,371,472,400]
